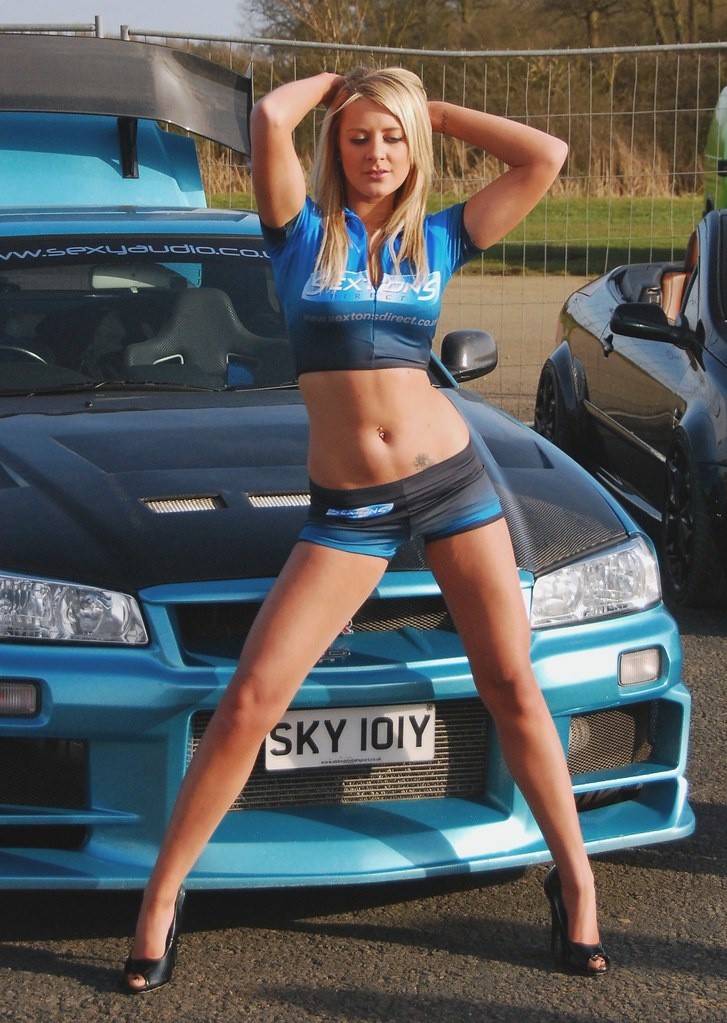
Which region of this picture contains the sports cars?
[530,210,727,617]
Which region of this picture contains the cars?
[0,207,696,893]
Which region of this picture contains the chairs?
[0,309,98,385]
[660,231,698,322]
[125,288,297,387]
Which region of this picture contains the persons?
[123,68,612,994]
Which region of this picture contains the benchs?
[0,288,254,387]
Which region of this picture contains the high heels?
[544,864,610,979]
[121,885,187,992]
